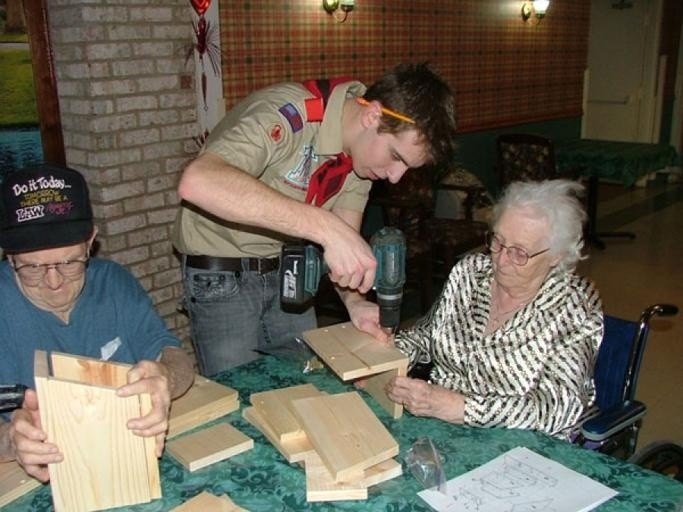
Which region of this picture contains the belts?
[181,256,283,274]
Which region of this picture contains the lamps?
[323,0,354,24]
[521,1,551,27]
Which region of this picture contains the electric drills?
[280,226,407,348]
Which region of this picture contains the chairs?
[365,131,556,318]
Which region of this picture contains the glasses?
[482,230,551,266]
[13,249,90,278]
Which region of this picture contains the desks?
[0,344,683,510]
[559,138,674,251]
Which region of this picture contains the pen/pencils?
[356,97,416,124]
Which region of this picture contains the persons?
[169,61,458,378]
[0,167,196,483]
[352,178,606,444]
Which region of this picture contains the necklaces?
[491,279,534,327]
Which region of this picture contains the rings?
[401,397,407,405]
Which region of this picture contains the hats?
[1,166,94,251]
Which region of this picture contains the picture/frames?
[1,2,65,185]
